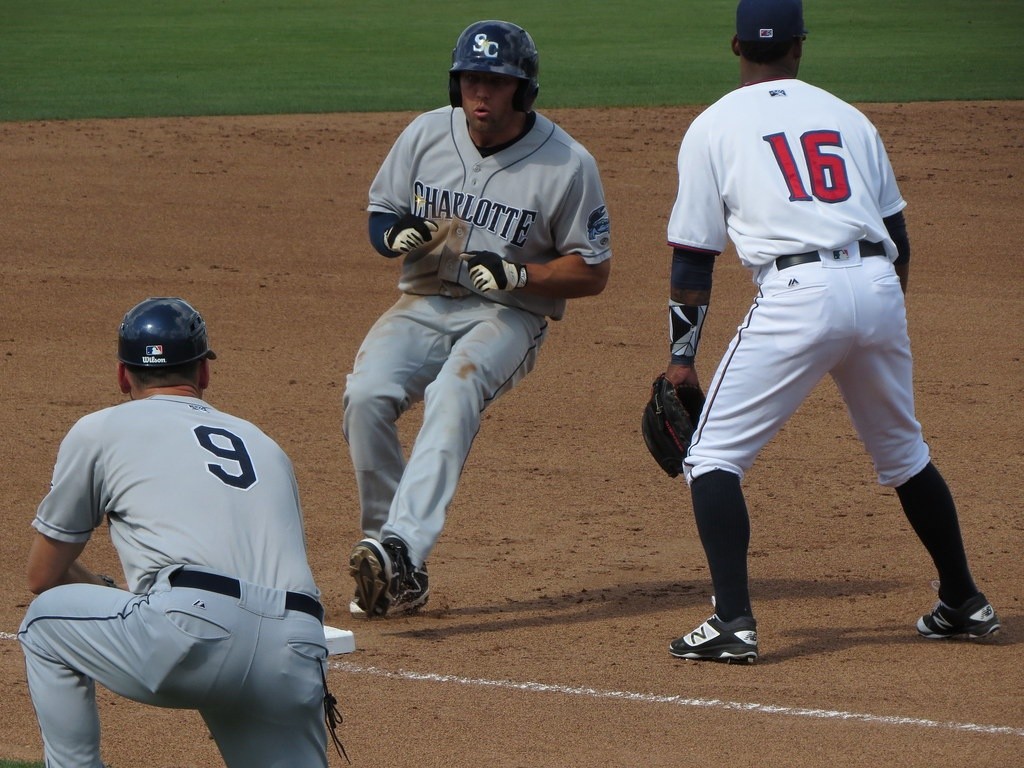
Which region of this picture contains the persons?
[662,0,1001,664]
[17,297,330,768]
[341,21,614,621]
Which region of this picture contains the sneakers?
[668,595,759,662]
[914,580,1001,640]
[348,538,430,619]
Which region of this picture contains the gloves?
[459,250,528,293]
[383,213,439,254]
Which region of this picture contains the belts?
[775,240,888,272]
[168,569,326,625]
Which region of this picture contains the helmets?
[117,296,218,368]
[446,20,541,114]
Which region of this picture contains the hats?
[735,0,809,41]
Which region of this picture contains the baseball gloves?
[639,374,708,478]
